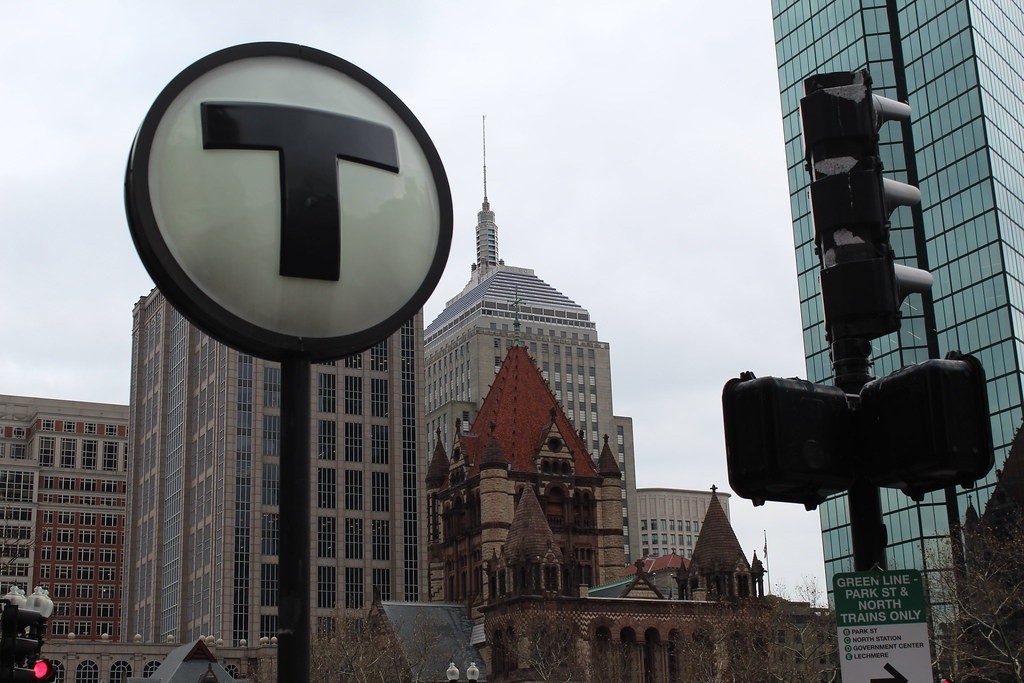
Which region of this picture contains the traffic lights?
[801,71,933,340]
[0,605,41,683]
[26,658,53,682]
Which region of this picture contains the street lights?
[2,585,53,668]
